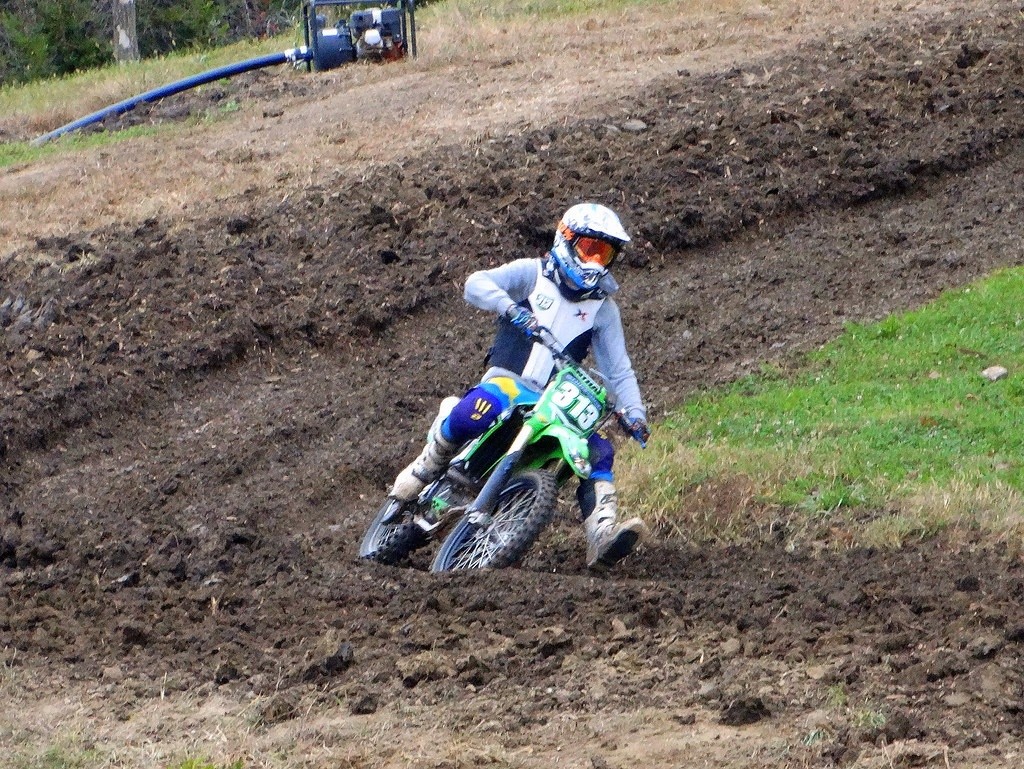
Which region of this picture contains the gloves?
[618,411,652,445]
[509,306,540,339]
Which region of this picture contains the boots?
[574,478,647,573]
[388,430,461,504]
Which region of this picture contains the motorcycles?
[355,304,653,574]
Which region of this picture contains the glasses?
[558,220,623,275]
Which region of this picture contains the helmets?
[553,202,630,302]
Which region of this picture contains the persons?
[388,202,654,570]
